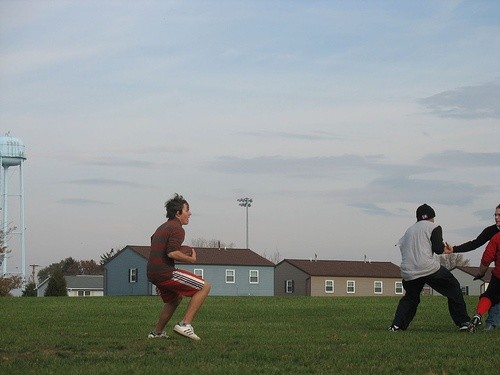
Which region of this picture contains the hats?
[416,204,435,221]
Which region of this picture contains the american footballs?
[175,246,193,262]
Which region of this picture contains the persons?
[147,192,210,340]
[388,203,471,331]
[444,203,500,329]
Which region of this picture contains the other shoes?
[483,324,494,331]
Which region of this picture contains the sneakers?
[148,331,170,339]
[173,321,200,341]
[388,325,401,332]
[471,314,482,326]
[459,321,476,333]
